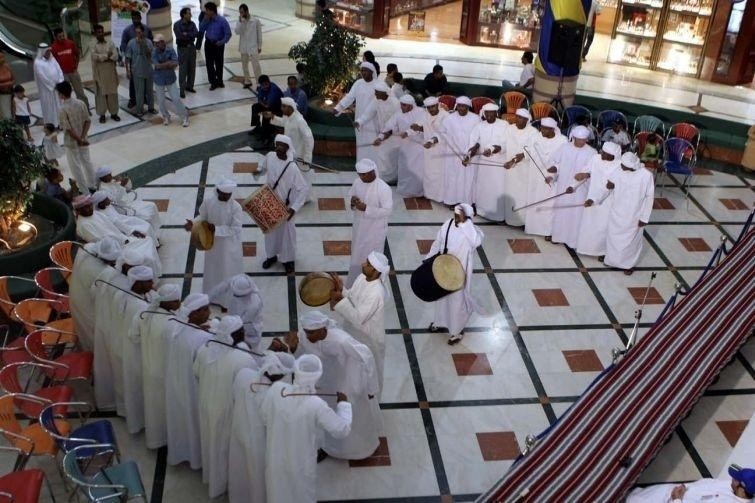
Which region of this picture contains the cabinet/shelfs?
[606,0,718,79]
[326,0,390,39]
[459,0,544,53]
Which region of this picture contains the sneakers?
[128,98,136,108]
[99,115,105,123]
[164,117,168,125]
[282,261,294,273]
[153,110,158,114]
[180,87,185,98]
[186,88,196,93]
[429,323,439,333]
[448,330,464,345]
[263,256,277,268]
[183,117,190,127]
[244,84,252,88]
[137,113,143,116]
[111,114,120,121]
[210,84,224,90]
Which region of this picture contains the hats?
[153,34,164,42]
[729,464,755,494]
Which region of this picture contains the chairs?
[436,91,701,199]
[0,446,57,503]
[0,240,98,410]
[39,401,122,503]
[0,393,70,493]
[0,361,86,426]
[62,443,148,503]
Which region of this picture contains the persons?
[35,42,64,131]
[122,11,154,108]
[331,50,655,275]
[250,73,314,207]
[421,203,485,344]
[151,34,190,127]
[201,2,232,90]
[0,50,16,121]
[174,7,198,98]
[625,464,755,503]
[69,166,353,503]
[11,84,34,142]
[41,123,59,166]
[195,0,220,51]
[90,23,120,123]
[46,168,79,204]
[235,4,262,88]
[285,250,392,459]
[124,24,159,117]
[50,28,92,115]
[54,81,95,195]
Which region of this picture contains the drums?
[193,221,214,250]
[410,254,465,302]
[299,272,337,306]
[240,184,290,233]
